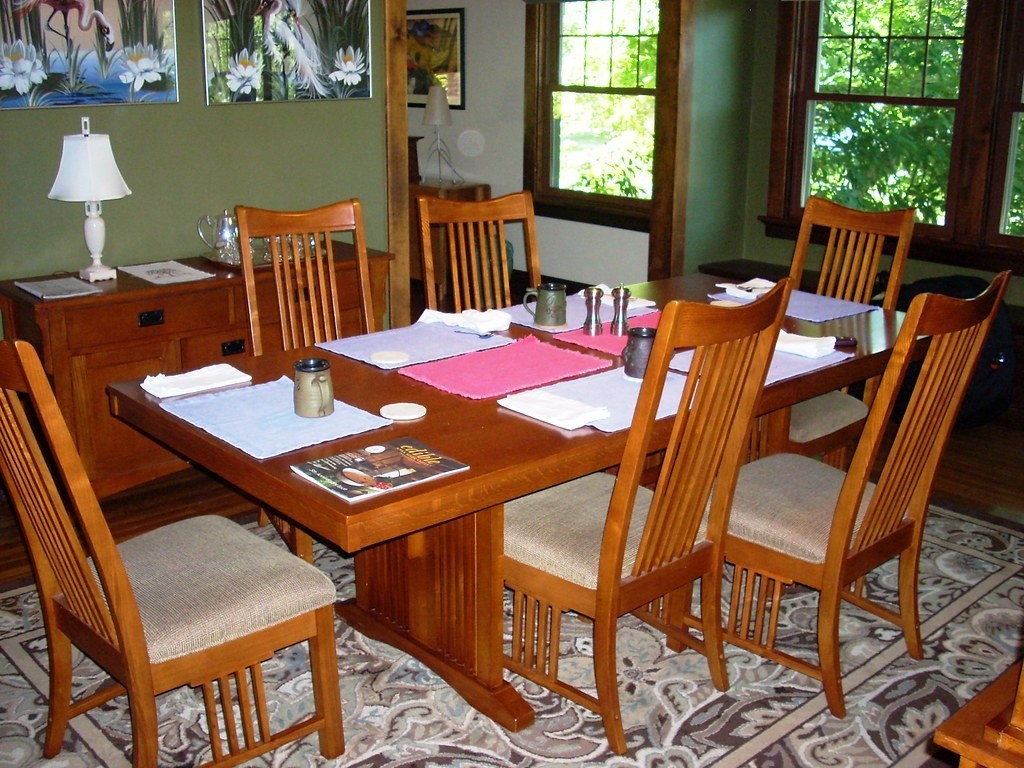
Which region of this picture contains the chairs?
[0,195,1010,768]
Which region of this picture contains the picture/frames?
[404,8,467,111]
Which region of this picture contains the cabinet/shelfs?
[410,179,491,304]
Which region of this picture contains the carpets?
[2,476,1024,768]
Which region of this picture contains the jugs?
[196,209,240,249]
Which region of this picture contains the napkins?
[143,360,251,401]
[714,278,777,299]
[775,328,836,358]
[576,284,656,309]
[497,390,609,431]
[420,307,513,334]
[162,379,392,462]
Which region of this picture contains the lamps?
[45,115,131,285]
[423,84,465,185]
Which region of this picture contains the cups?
[214,233,317,263]
[294,357,335,418]
[622,327,657,378]
[523,282,567,326]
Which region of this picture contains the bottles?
[582,288,604,336]
[611,283,632,336]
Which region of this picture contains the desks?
[99,271,917,735]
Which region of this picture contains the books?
[290,437,470,502]
[15,277,103,299]
